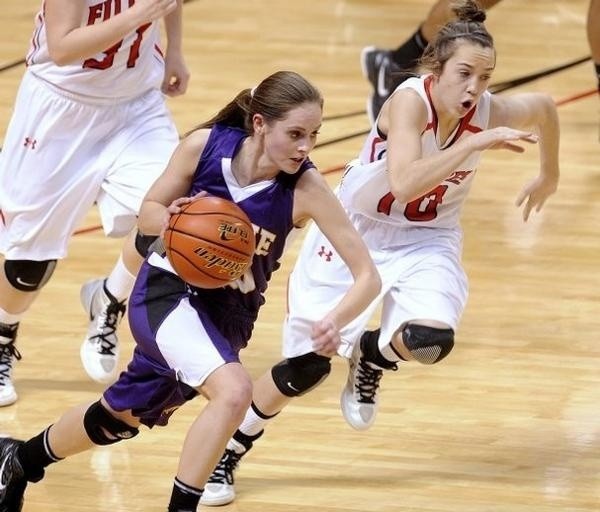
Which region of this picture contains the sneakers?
[339,329,399,433]
[360,46,416,130]
[0,437,46,512]
[197,432,253,508]
[0,321,22,408]
[80,277,129,386]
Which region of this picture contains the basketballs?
[164,196,255,290]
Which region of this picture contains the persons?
[193,2,559,506]
[362,0,599,129]
[0,70,382,511]
[0,2,190,406]
